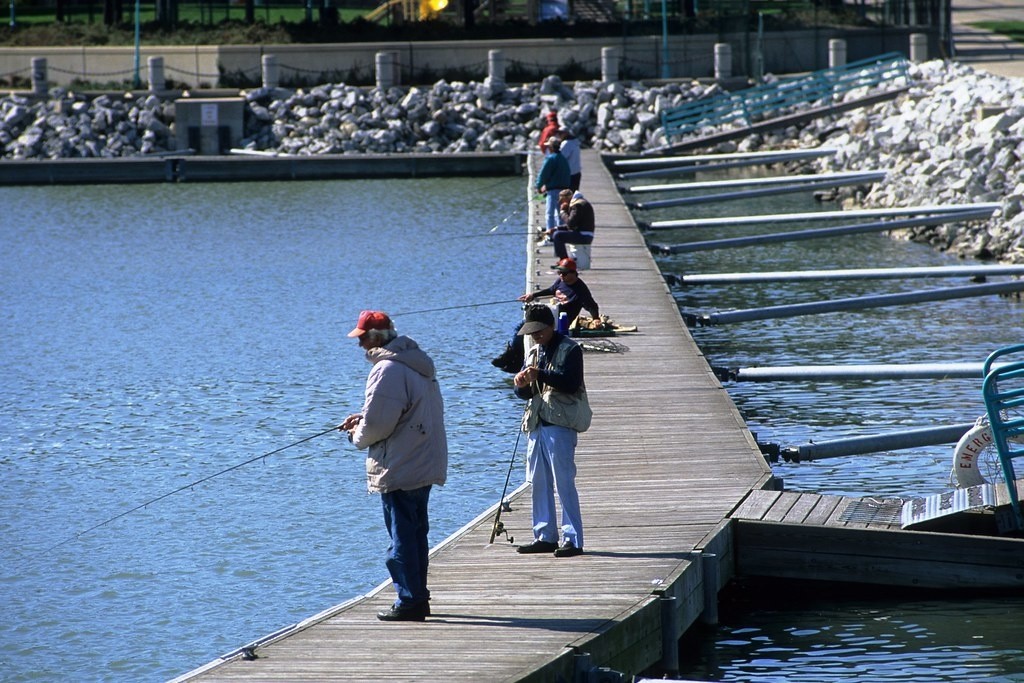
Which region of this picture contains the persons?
[491,256,599,374]
[513,304,593,557]
[547,189,595,269]
[535,136,571,247]
[338,311,447,621]
[540,112,582,192]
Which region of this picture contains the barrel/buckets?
[569,244,591,270]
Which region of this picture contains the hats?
[556,259,577,260]
[557,189,573,203]
[545,113,556,121]
[543,137,561,146]
[518,305,556,334]
[555,126,569,132]
[348,310,395,337]
[556,258,576,271]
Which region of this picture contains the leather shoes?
[377,607,425,622]
[391,603,431,616]
[516,539,558,553]
[554,541,583,556]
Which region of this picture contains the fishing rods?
[40,417,361,557]
[488,401,528,546]
[331,299,531,325]
[473,172,536,193]
[465,194,540,251]
[441,232,549,242]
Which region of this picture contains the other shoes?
[537,240,553,247]
[551,260,563,269]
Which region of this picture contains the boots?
[492,340,524,374]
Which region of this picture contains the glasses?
[557,271,566,274]
